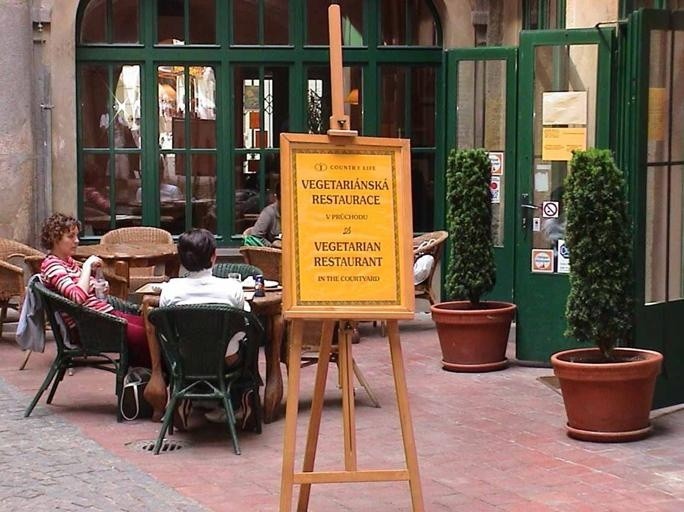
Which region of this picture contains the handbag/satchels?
[120,365,154,422]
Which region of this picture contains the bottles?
[254,274,264,297]
[94,261,106,301]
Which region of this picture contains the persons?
[248,178,282,246]
[158,225,251,432]
[542,186,567,261]
[137,162,182,201]
[239,172,271,215]
[83,166,113,209]
[37,211,168,384]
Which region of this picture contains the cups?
[228,273,242,283]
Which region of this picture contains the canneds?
[256,275,265,296]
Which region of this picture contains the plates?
[242,281,282,292]
[134,280,164,295]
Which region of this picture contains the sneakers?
[171,396,193,432]
[240,389,260,431]
[204,405,244,423]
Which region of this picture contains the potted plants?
[549,144,665,445]
[428,144,518,372]
